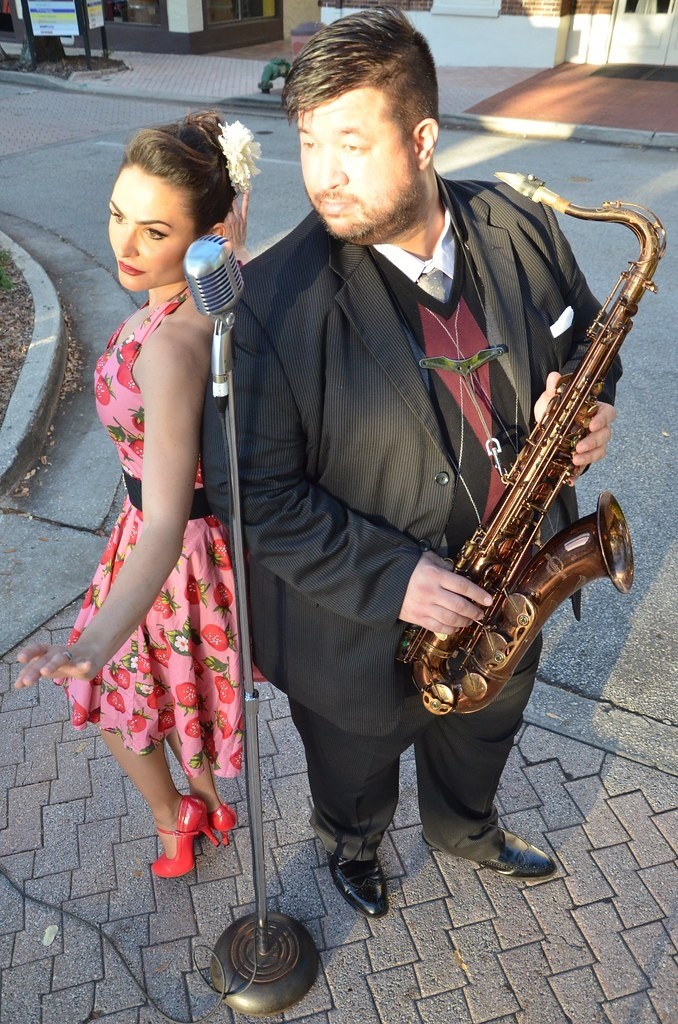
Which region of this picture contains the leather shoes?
[327,852,388,918]
[422,829,557,879]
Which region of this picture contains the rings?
[63,651,73,662]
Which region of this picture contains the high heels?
[152,795,220,877]
[207,803,238,846]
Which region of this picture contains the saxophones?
[399,167,667,718]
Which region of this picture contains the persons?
[203,8,622,920]
[14,112,261,878]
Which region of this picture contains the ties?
[416,270,448,304]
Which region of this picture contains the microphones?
[184,235,244,415]
